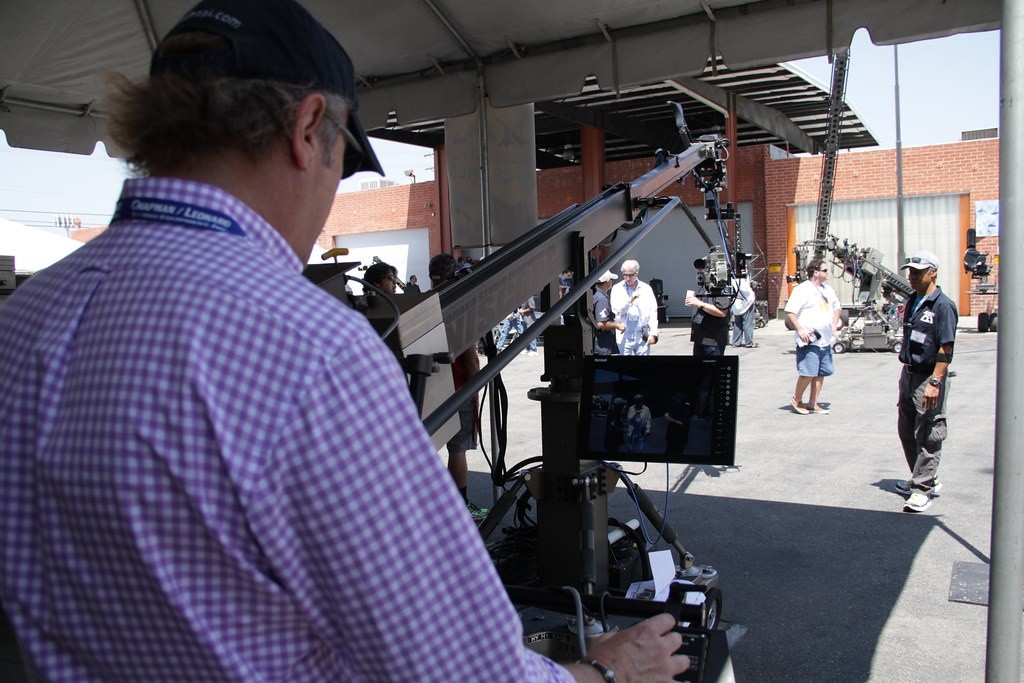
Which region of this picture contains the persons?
[895,250,959,512]
[361,253,842,415]
[0,1,691,683]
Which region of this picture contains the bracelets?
[700,303,706,310]
[576,658,617,683]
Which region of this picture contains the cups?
[685,289,695,307]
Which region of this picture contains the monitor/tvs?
[578,353,738,467]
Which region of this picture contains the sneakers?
[895,475,942,496]
[467,502,490,520]
[806,404,830,414]
[903,484,935,512]
[528,350,539,357]
[746,342,760,348]
[790,397,809,414]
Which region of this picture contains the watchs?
[930,378,942,386]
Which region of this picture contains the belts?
[905,364,928,374]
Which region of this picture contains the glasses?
[322,113,364,180]
[905,257,936,267]
[623,272,636,277]
[817,269,828,273]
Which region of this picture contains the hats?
[693,256,708,269]
[596,270,618,283]
[151,0,388,177]
[900,256,938,270]
